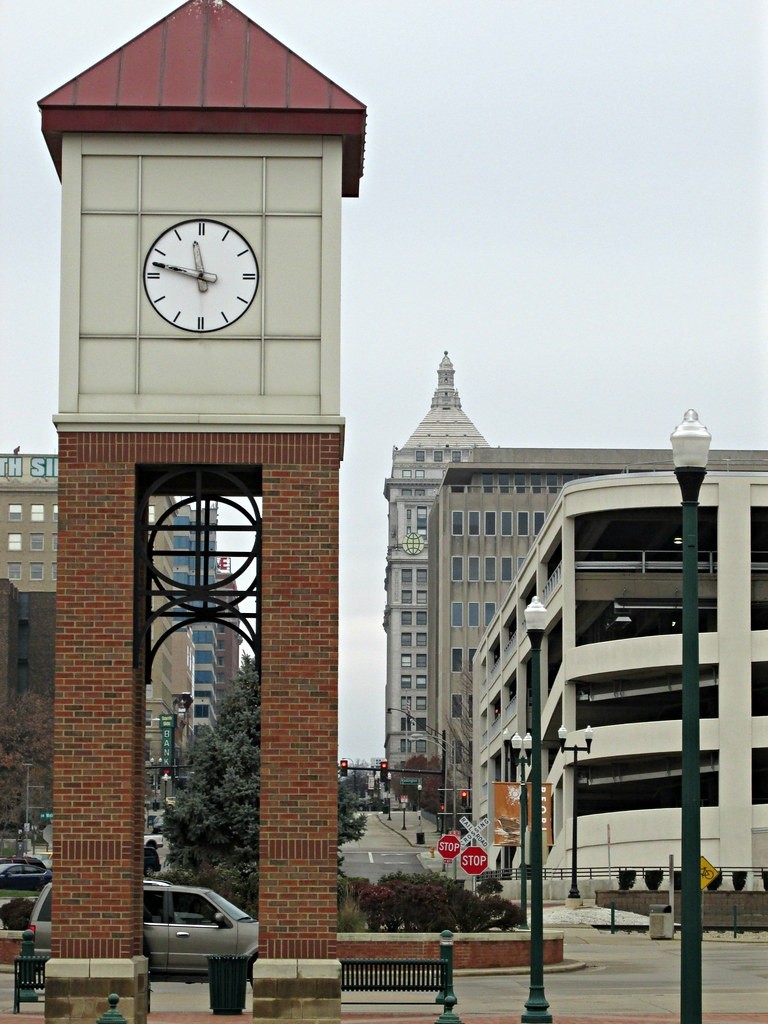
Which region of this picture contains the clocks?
[143,219,262,333]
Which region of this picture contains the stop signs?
[437,834,461,859]
[461,845,489,875]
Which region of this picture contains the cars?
[143,834,165,851]
[142,846,162,878]
[0,864,52,891]
[28,878,260,989]
[34,851,53,868]
[0,857,47,869]
[165,796,177,811]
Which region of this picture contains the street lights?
[518,594,553,1024]
[387,707,445,833]
[511,731,534,929]
[558,724,593,898]
[149,756,163,797]
[409,737,447,873]
[668,409,713,1024]
[409,734,458,880]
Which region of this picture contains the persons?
[186,898,205,925]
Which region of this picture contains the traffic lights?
[462,791,467,806]
[380,761,388,779]
[438,785,445,811]
[340,760,348,776]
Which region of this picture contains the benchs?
[12,956,49,1015]
[339,958,450,1011]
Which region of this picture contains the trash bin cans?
[382,805,389,814]
[203,953,252,1015]
[650,903,676,940]
[416,832,425,845]
